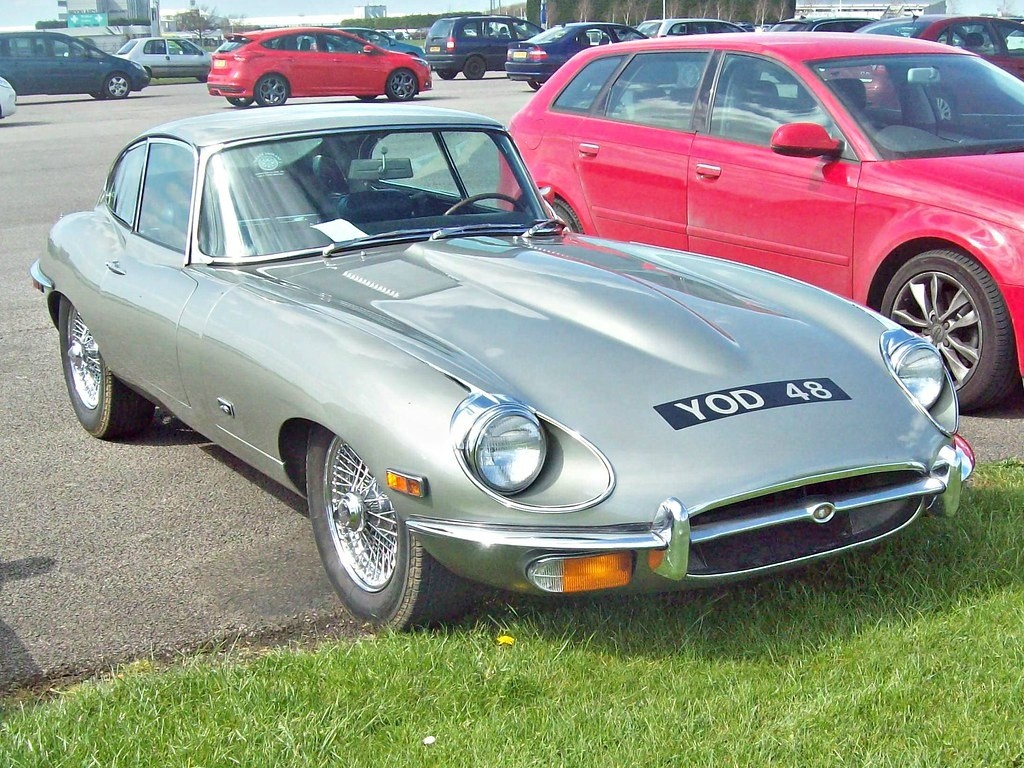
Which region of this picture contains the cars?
[423,14,546,80]
[204,25,433,108]
[585,12,1005,128]
[297,26,426,61]
[499,29,1023,417]
[28,100,978,640]
[0,31,150,100]
[110,36,213,83]
[0,77,17,121]
[504,21,678,93]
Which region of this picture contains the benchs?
[624,79,698,133]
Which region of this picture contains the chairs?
[300,39,311,51]
[599,37,609,45]
[826,77,867,112]
[498,26,510,39]
[679,26,685,33]
[336,189,413,224]
[964,33,984,54]
[724,80,781,148]
[489,27,497,38]
